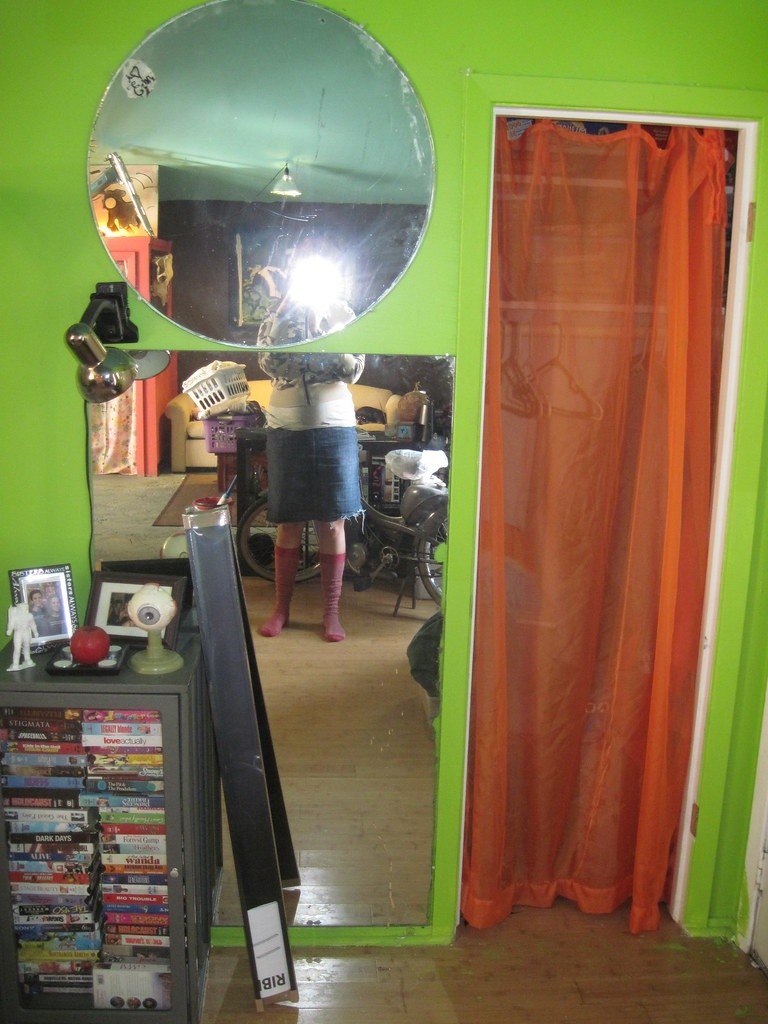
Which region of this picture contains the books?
[0,706,179,1012]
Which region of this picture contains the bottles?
[416,403,433,442]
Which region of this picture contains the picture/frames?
[8,563,81,657]
[84,570,187,653]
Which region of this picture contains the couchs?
[162,379,402,474]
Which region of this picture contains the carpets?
[152,470,315,529]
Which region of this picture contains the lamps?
[121,349,171,381]
[63,282,140,406]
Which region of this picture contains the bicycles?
[236,448,449,618]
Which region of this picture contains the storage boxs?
[201,413,255,454]
[181,363,251,415]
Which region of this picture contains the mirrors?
[77,349,459,936]
[86,0,437,355]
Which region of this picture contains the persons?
[28,590,49,638]
[109,599,125,624]
[47,595,66,634]
[257,256,363,643]
